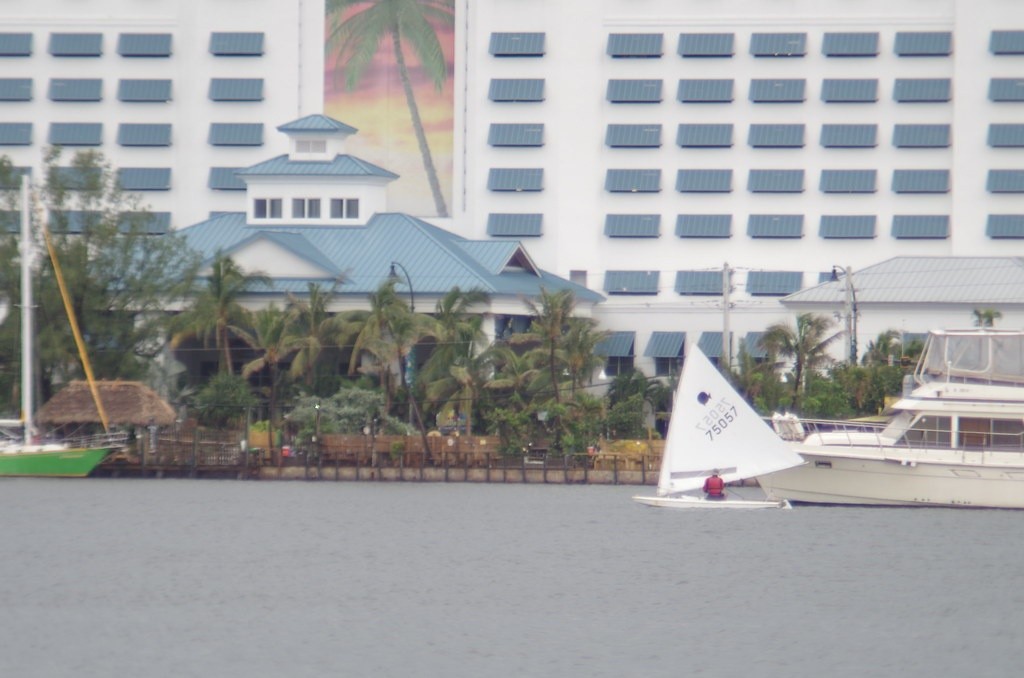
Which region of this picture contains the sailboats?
[0,174,123,477]
[630,344,793,515]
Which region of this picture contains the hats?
[712,469,718,476]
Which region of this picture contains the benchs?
[524,447,548,470]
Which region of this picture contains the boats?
[758,325,1024,514]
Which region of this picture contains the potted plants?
[249,420,283,463]
[293,426,322,467]
[389,441,406,466]
[588,440,596,454]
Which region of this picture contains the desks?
[571,453,631,470]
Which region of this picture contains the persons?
[703,468,725,501]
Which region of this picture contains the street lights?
[826,263,862,366]
[388,261,414,420]
